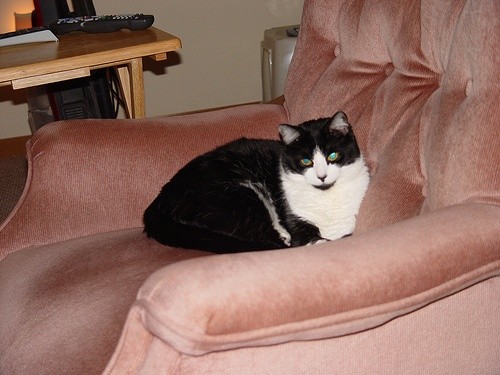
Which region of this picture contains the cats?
[141,111,370,255]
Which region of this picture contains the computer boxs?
[44,72,114,120]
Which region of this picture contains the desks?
[0,20,183,120]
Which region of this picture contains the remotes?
[51,14,154,32]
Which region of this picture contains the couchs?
[0,0,500,374]
